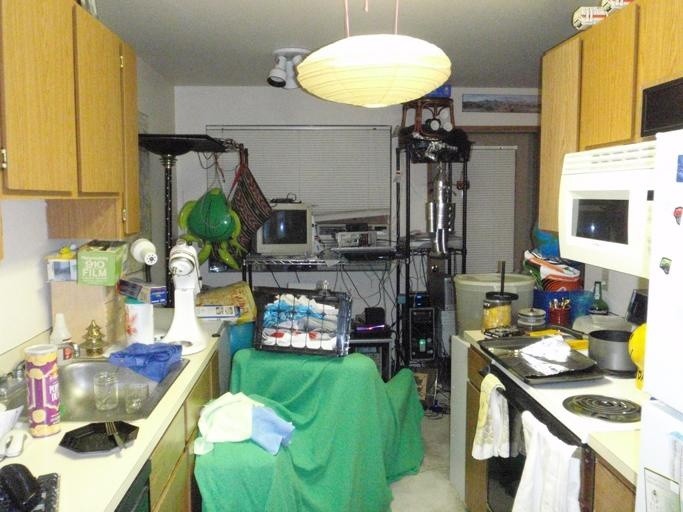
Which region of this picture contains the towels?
[512,411,581,512]
[510,405,526,459]
[471,374,510,462]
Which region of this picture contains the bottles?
[24,343,60,437]
[517,308,547,336]
[123,297,154,347]
[586,281,609,314]
[47,312,75,366]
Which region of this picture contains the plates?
[57,419,139,459]
[543,278,582,293]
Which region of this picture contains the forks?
[105,421,128,452]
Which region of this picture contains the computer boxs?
[401,305,436,362]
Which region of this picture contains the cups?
[548,306,571,329]
[92,372,118,410]
[124,381,149,414]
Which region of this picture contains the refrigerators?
[634,129,683,512]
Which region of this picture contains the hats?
[194,390,264,457]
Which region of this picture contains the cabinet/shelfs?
[47,43,139,242]
[449,330,478,501]
[115,328,231,512]
[395,146,469,373]
[1,0,124,199]
[579,1,682,152]
[538,36,580,234]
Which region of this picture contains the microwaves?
[558,139,657,280]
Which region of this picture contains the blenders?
[160,238,211,356]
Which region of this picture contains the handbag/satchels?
[111,343,181,383]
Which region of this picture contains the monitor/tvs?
[252,200,313,259]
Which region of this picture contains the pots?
[546,324,640,376]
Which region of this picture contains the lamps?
[267,47,310,89]
[297,0,453,109]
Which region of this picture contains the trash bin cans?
[454,272,537,337]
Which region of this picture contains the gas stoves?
[491,349,644,443]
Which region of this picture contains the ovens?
[478,365,595,511]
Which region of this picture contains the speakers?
[365,306,385,325]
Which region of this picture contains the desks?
[350,328,392,384]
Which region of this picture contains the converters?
[345,222,368,230]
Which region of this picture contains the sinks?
[9,351,190,421]
